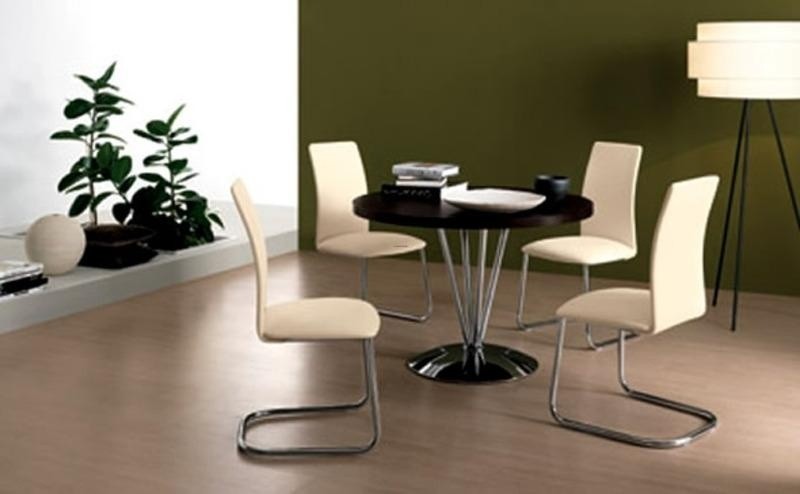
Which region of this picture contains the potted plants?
[49,58,225,271]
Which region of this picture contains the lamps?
[684,18,799,331]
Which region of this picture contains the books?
[379,161,469,207]
[1,259,49,303]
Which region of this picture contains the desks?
[354,188,594,387]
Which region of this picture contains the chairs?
[232,178,382,458]
[309,140,433,323]
[520,140,643,350]
[548,175,719,449]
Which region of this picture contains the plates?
[443,187,547,214]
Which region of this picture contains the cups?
[535,173,570,202]
